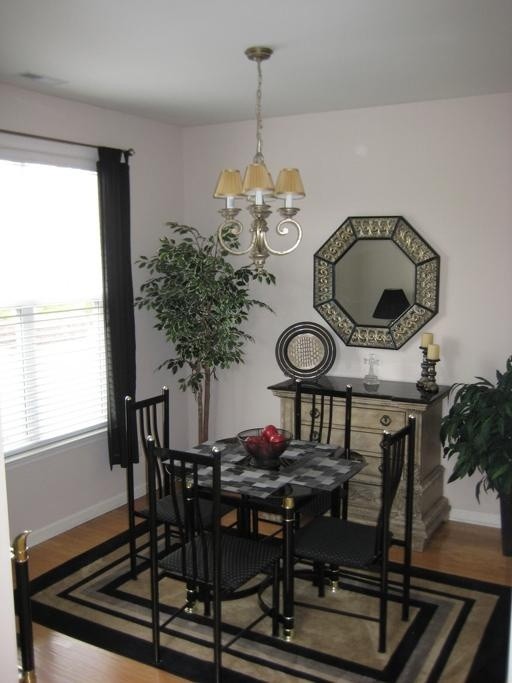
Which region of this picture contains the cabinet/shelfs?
[267,375,451,553]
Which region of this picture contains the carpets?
[14,518,512,683]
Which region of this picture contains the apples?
[245,425,287,456]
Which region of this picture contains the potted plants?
[438,355,512,556]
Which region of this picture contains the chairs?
[254,379,353,565]
[145,434,280,683]
[124,384,242,604]
[280,413,416,653]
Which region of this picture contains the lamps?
[372,288,410,319]
[213,47,306,271]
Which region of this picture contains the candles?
[422,333,433,348]
[427,344,439,360]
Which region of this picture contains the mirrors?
[313,216,441,350]
